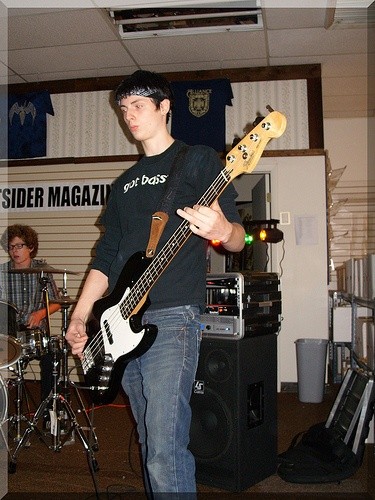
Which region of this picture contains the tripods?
[9,272,99,472]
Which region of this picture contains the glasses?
[8,243,28,252]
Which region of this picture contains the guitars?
[80,107,288,404]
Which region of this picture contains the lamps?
[259,226,284,244]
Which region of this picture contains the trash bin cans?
[295,338,328,404]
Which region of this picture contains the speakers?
[186,334,278,492]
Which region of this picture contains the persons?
[0,224,69,435]
[65,70,245,500]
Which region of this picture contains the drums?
[0,377,9,427]
[18,330,48,357]
[0,299,24,370]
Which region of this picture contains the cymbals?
[37,297,78,303]
[2,265,85,275]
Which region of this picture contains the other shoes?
[43,420,65,432]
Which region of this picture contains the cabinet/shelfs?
[328,291,375,444]
